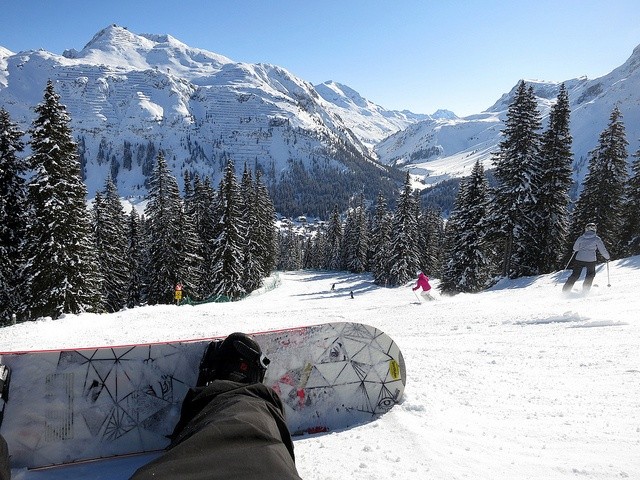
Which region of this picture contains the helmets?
[416,270,423,277]
[585,223,597,233]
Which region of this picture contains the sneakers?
[205,333,262,385]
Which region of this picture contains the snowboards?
[0,322,406,472]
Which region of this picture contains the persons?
[1,329,300,479]
[331,283,335,291]
[561,222,611,295]
[349,290,354,299]
[412,271,435,302]
[173,282,182,305]
